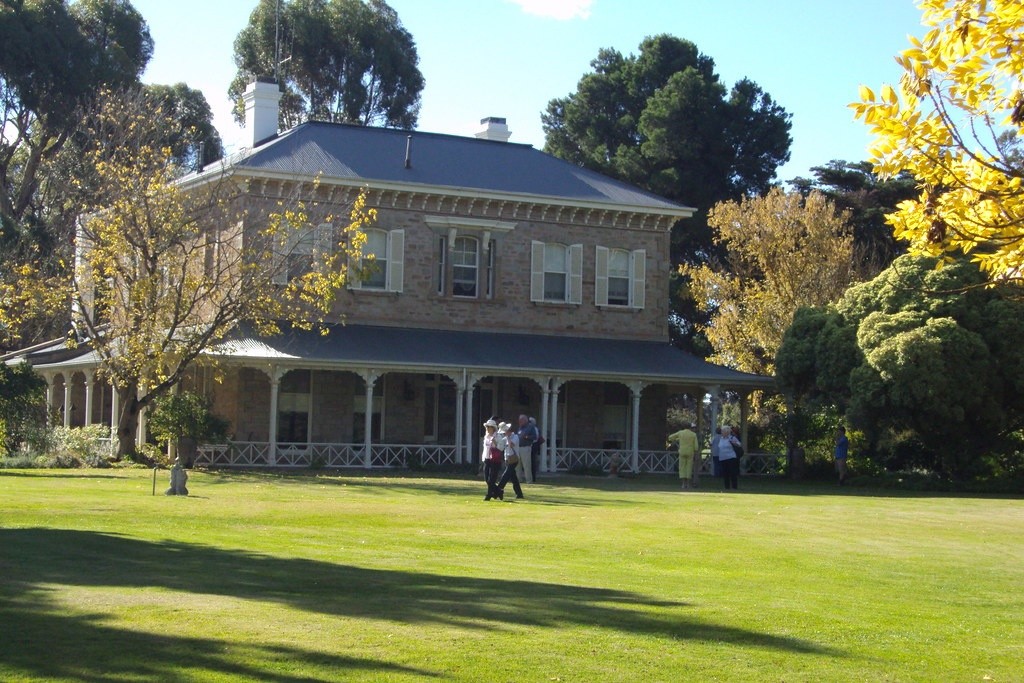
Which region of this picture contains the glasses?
[691,427,697,429]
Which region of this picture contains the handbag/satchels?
[533,436,545,446]
[489,444,502,464]
[731,435,744,458]
[508,455,518,464]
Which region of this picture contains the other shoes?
[492,493,498,498]
[498,490,503,500]
[485,495,490,500]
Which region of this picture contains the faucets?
[154,463,160,471]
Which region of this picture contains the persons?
[668,421,704,489]
[710,428,721,476]
[481,416,524,501]
[516,414,545,484]
[835,427,848,487]
[718,426,740,488]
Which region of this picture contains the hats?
[496,421,512,433]
[483,419,498,431]
